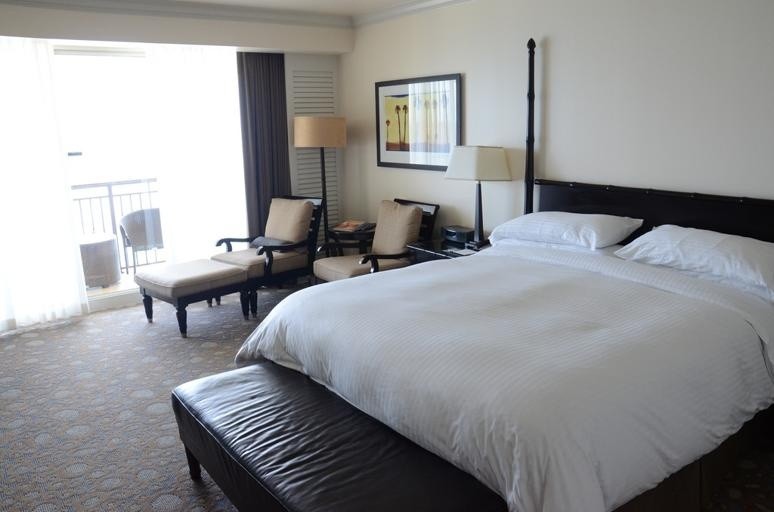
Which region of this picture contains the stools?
[135,258,249,337]
[79,233,119,288]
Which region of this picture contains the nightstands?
[407,237,489,266]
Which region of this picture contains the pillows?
[492,210,644,251]
[614,223,774,289]
[249,236,293,253]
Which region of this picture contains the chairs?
[119,208,162,274]
[211,195,325,317]
[310,197,440,286]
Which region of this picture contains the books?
[333,220,366,232]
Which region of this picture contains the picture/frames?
[374,73,461,171]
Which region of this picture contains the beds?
[236,37,774,508]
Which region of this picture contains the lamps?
[444,145,511,249]
[294,114,346,256]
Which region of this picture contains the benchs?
[170,363,509,510]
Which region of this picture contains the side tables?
[327,224,376,255]
